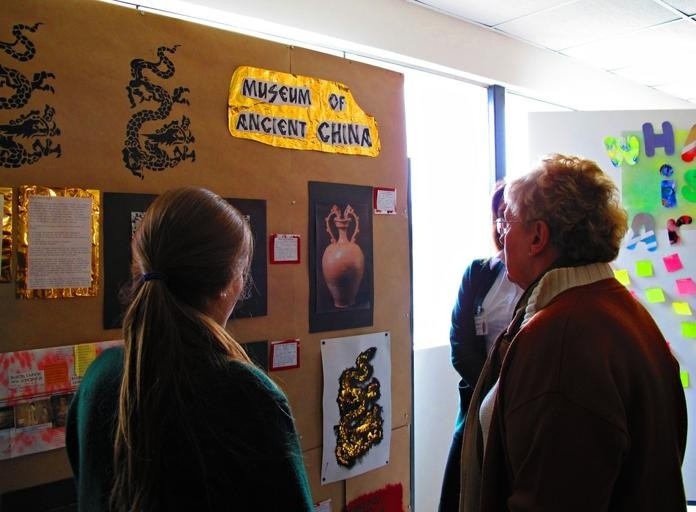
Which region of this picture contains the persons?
[459,152,688,506]
[57,398,69,426]
[39,404,51,426]
[63,185,316,508]
[24,399,40,427]
[437,179,528,506]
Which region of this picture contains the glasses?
[495,217,527,234]
